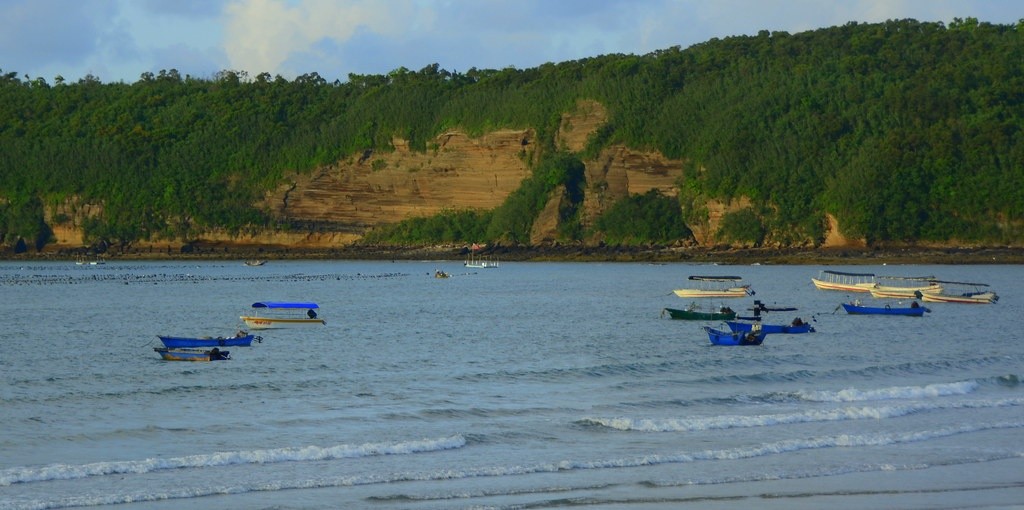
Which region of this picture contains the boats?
[240,300,328,331]
[868,274,945,300]
[672,282,756,298]
[244,259,268,267]
[664,306,737,320]
[725,319,817,334]
[702,325,767,347]
[153,347,232,362]
[840,294,932,318]
[687,275,743,282]
[913,277,1000,305]
[811,270,877,293]
[736,316,762,321]
[156,327,264,349]
